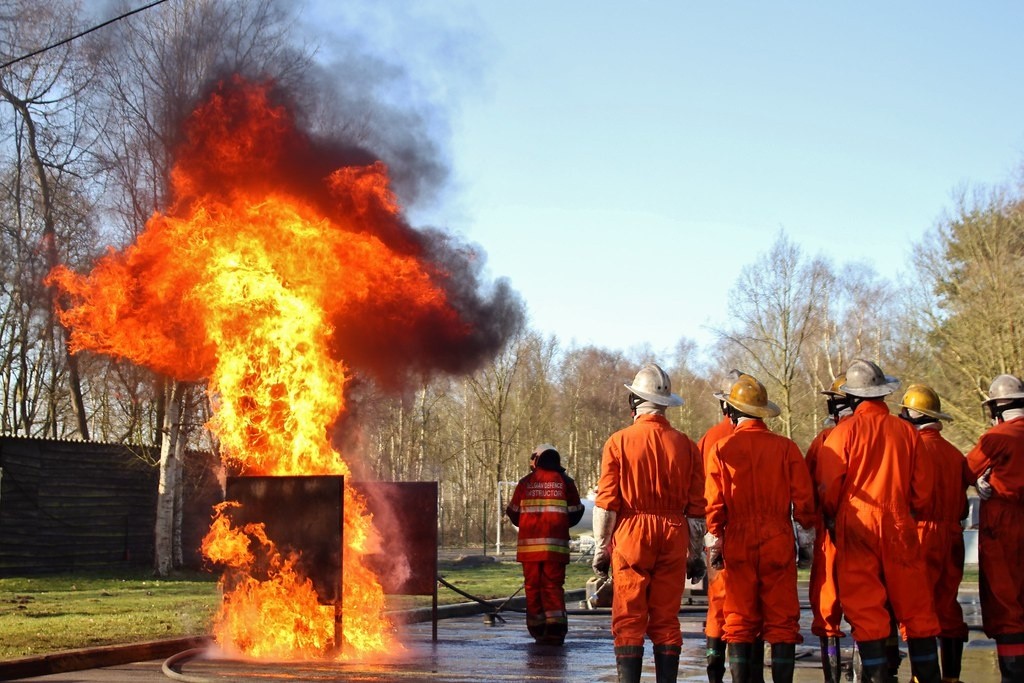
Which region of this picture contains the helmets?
[713,369,744,401]
[839,358,903,398]
[726,373,781,418]
[980,375,1024,406]
[820,373,849,398]
[898,383,955,422]
[531,443,556,457]
[623,363,684,406]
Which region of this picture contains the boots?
[857,639,888,683]
[819,635,842,683]
[907,636,937,683]
[653,643,682,683]
[998,653,1024,683]
[754,639,766,683]
[728,642,754,683]
[771,642,795,683]
[614,646,644,683]
[706,636,727,683]
[940,639,966,683]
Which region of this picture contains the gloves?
[975,467,992,499]
[687,517,709,585]
[710,547,725,572]
[796,525,815,570]
[592,504,617,582]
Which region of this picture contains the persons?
[804,359,970,683]
[965,374,1024,683]
[698,358,816,683]
[506,444,585,640]
[591,364,708,683]
